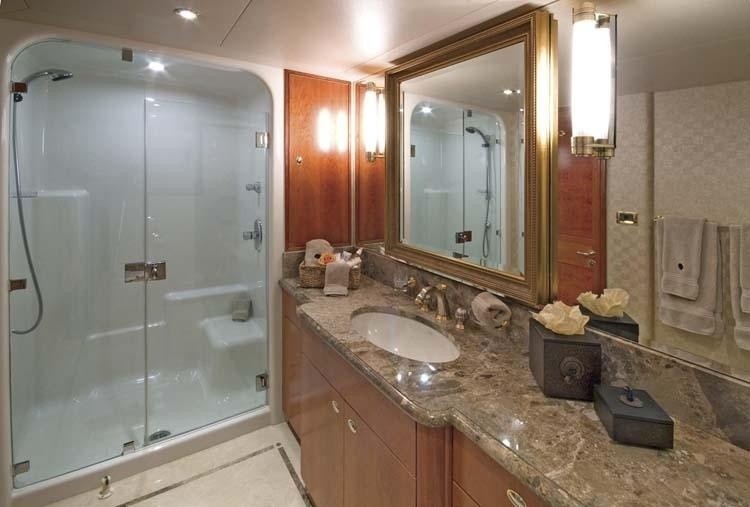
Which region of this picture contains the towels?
[655,213,750,352]
[322,260,351,297]
[470,289,513,339]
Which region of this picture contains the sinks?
[349,306,461,363]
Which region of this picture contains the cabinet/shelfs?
[448,430,550,505]
[296,355,418,507]
[283,315,302,448]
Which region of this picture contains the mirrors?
[380,10,558,315]
[557,0,747,386]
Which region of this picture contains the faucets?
[416,285,453,322]
